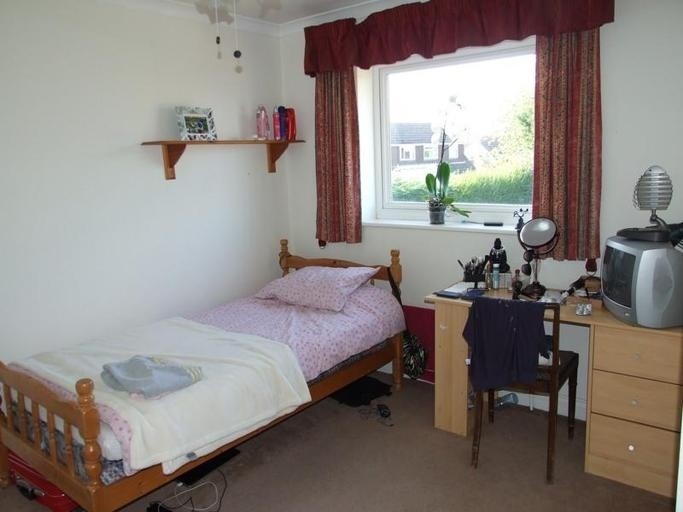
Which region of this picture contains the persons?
[184,116,207,133]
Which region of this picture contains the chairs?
[466,296,582,488]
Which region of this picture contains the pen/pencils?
[457,256,490,274]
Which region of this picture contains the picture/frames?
[175,104,218,141]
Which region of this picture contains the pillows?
[253,264,382,313]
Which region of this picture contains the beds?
[0,239,407,511]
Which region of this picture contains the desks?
[423,282,683,502]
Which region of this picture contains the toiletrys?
[255,104,296,142]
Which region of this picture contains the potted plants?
[425,162,472,225]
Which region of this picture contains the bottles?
[492,264,500,290]
[254,104,297,141]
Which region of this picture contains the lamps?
[516,216,561,300]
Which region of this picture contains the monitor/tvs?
[601,236,683,329]
